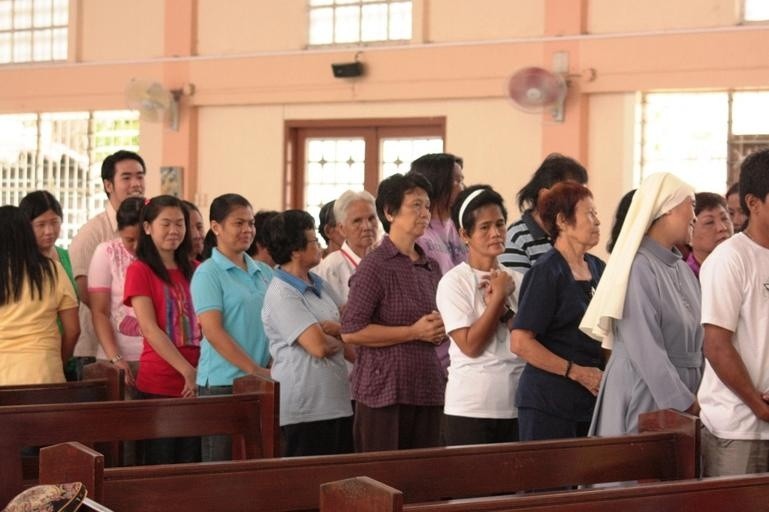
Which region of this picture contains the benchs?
[38,408,768,511]
[0,360,281,512]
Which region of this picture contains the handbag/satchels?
[4,481,94,511]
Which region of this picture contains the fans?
[506,46,598,124]
[121,71,197,131]
